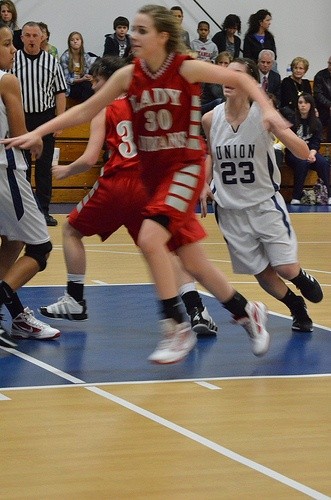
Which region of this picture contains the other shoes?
[291,199,300,204]
[328,197,331,204]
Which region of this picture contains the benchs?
[30,91,331,204]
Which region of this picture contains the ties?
[262,76,268,92]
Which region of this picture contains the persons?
[59,31,95,101]
[0,20,60,347]
[0,0,19,33]
[191,8,331,206]
[101,16,134,62]
[37,22,58,59]
[37,58,219,335]
[2,21,68,226]
[200,58,324,332]
[0,4,290,365]
[170,5,193,51]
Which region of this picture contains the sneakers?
[290,296,313,332]
[45,214,57,226]
[191,304,218,335]
[300,270,323,303]
[10,306,61,340]
[228,301,271,357]
[37,289,89,322]
[146,318,199,365]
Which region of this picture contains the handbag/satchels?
[301,182,327,206]
